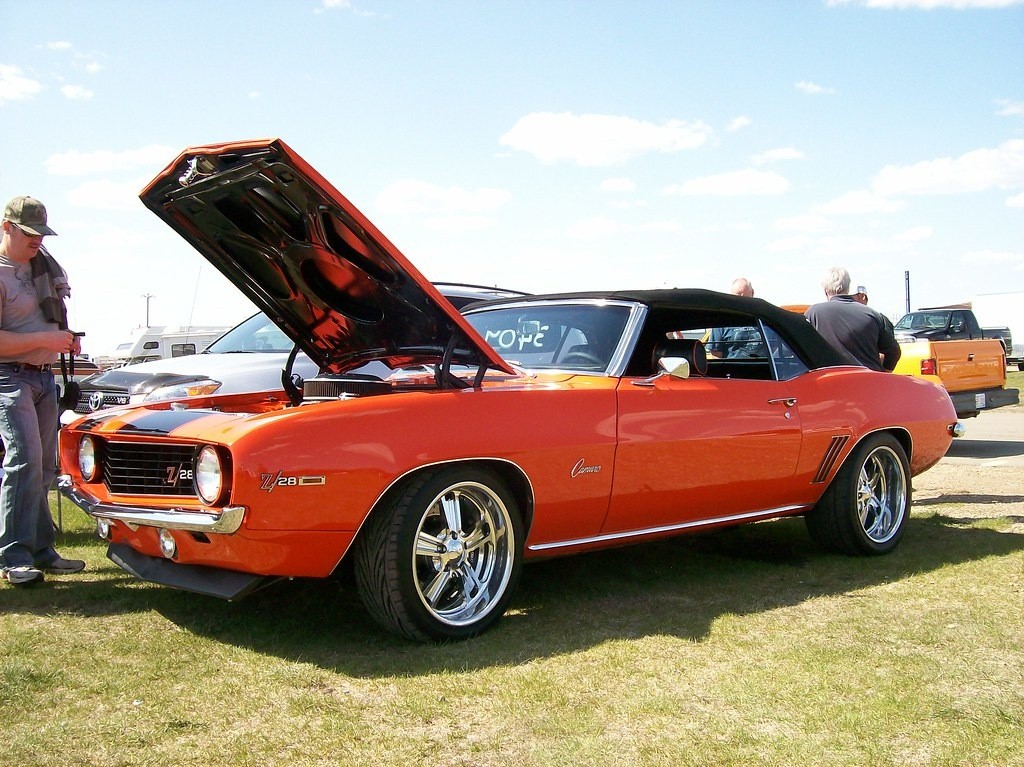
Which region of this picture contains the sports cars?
[56,136,966,644]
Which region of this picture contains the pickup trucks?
[892,306,1012,358]
[700,303,1020,420]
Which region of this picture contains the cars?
[58,279,588,429]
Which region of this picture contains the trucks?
[51,323,292,404]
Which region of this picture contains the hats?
[848,285,866,296]
[5,196,58,236]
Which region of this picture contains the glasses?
[9,222,36,237]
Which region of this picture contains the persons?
[772,269,901,373]
[707,278,777,358]
[0,196,86,585]
[849,285,894,365]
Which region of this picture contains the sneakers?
[45,558,86,573]
[0,565,44,586]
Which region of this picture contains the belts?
[6,362,52,373]
